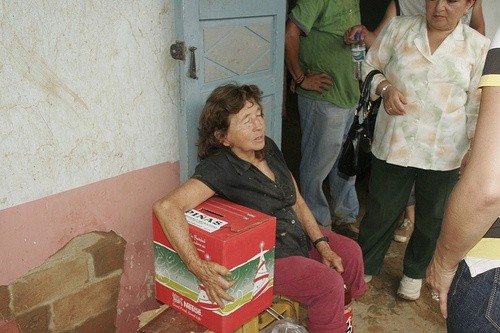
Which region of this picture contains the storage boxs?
[152,201,276,333]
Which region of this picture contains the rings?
[388,107,392,110]
[430,291,439,302]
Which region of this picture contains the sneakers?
[398,274,422,301]
[344,274,374,288]
[393,217,415,242]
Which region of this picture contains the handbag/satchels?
[338,70,386,177]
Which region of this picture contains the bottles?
[351,33,366,80]
[331,266,353,333]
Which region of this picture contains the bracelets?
[312,235,330,248]
[380,84,392,97]
[291,73,304,93]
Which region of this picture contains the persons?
[425,28,500,333]
[360,0,490,301]
[152,83,367,333]
[285,0,367,236]
[343,0,486,242]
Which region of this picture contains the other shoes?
[334,225,360,240]
